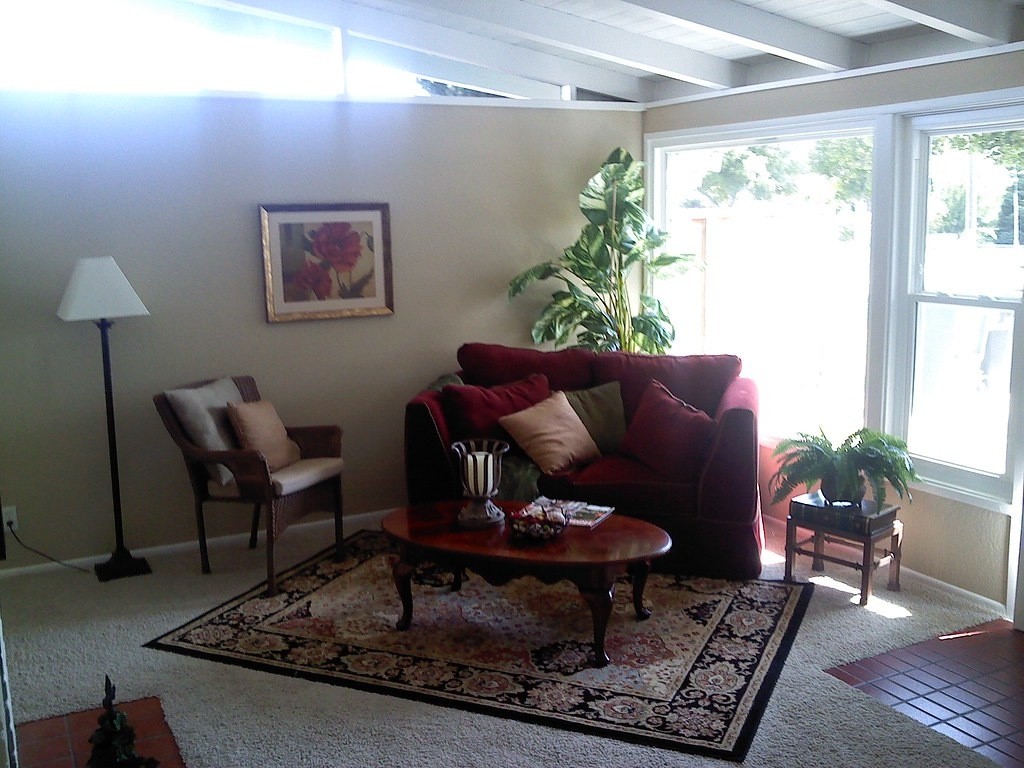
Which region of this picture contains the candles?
[467,452,495,496]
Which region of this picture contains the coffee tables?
[381,498,673,668]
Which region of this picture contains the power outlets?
[2,505,19,532]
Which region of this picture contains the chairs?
[153,374,346,598]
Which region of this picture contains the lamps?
[55,256,156,583]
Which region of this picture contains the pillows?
[164,374,246,487]
[498,389,603,477]
[441,373,551,460]
[227,398,302,476]
[568,379,627,455]
[619,376,720,484]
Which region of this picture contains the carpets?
[142,527,816,765]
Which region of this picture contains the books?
[518,495,616,527]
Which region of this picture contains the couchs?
[402,342,766,579]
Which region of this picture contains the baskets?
[506,498,574,543]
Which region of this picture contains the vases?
[450,437,511,524]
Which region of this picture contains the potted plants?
[768,423,927,517]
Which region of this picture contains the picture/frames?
[258,202,395,323]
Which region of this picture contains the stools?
[784,515,904,603]
